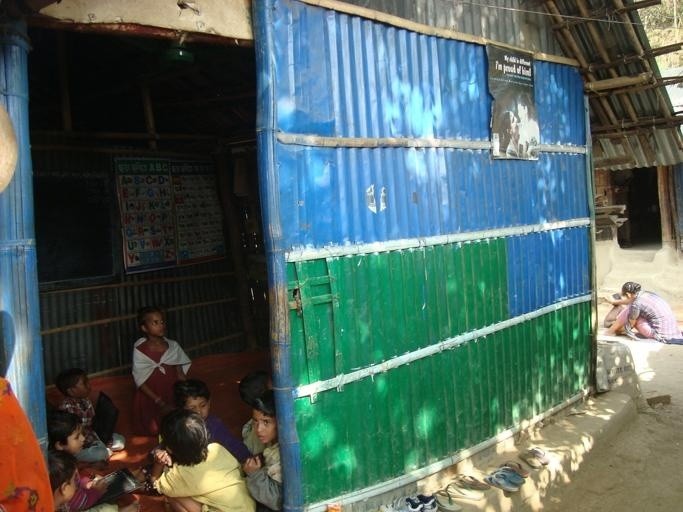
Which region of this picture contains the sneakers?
[380,493,438,512]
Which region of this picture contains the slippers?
[432,474,491,511]
[519,446,549,468]
[484,460,529,493]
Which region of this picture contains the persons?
[515,94,539,159]
[601,281,682,344]
[129,306,193,436]
[498,110,523,157]
[46,367,283,510]
